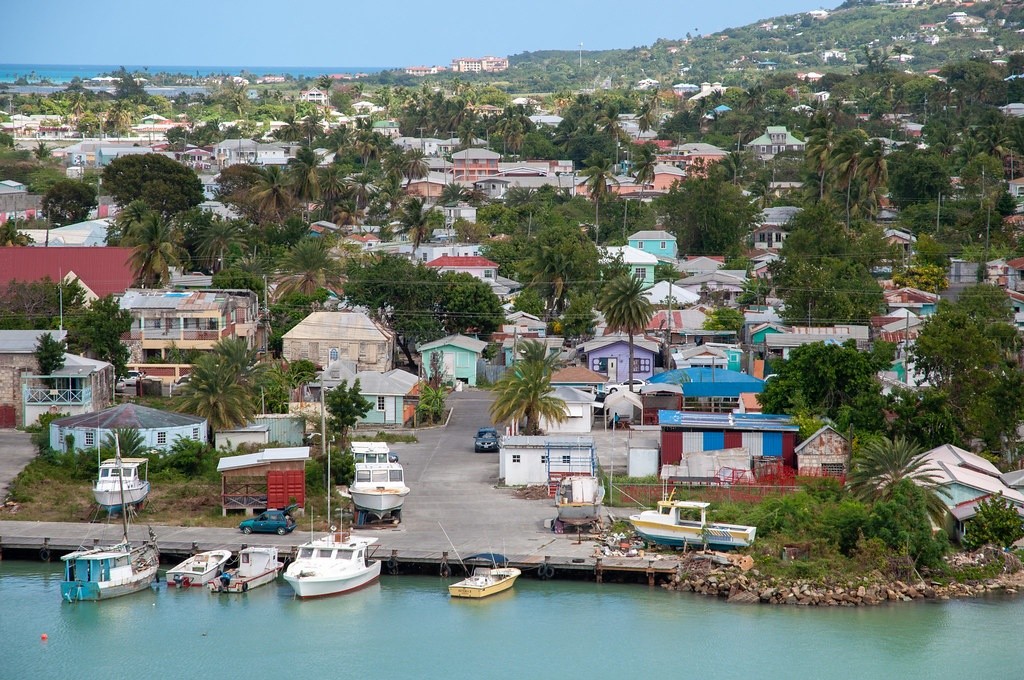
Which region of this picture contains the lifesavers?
[242,582,248,591]
[39,549,50,561]
[387,559,396,569]
[537,562,556,580]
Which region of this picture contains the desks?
[619,418,630,430]
[621,414,630,428]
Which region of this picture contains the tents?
[603,390,643,432]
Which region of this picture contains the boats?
[93,456,151,513]
[348,462,411,519]
[283,530,383,598]
[447,566,522,598]
[165,548,232,586]
[207,546,285,593]
[349,440,398,468]
[554,475,606,522]
[628,499,758,551]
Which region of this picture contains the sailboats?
[59,431,161,602]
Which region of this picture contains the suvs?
[473,426,500,452]
[239,503,299,535]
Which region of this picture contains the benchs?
[631,418,635,422]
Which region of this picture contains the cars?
[605,378,649,395]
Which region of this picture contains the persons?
[607,412,620,429]
